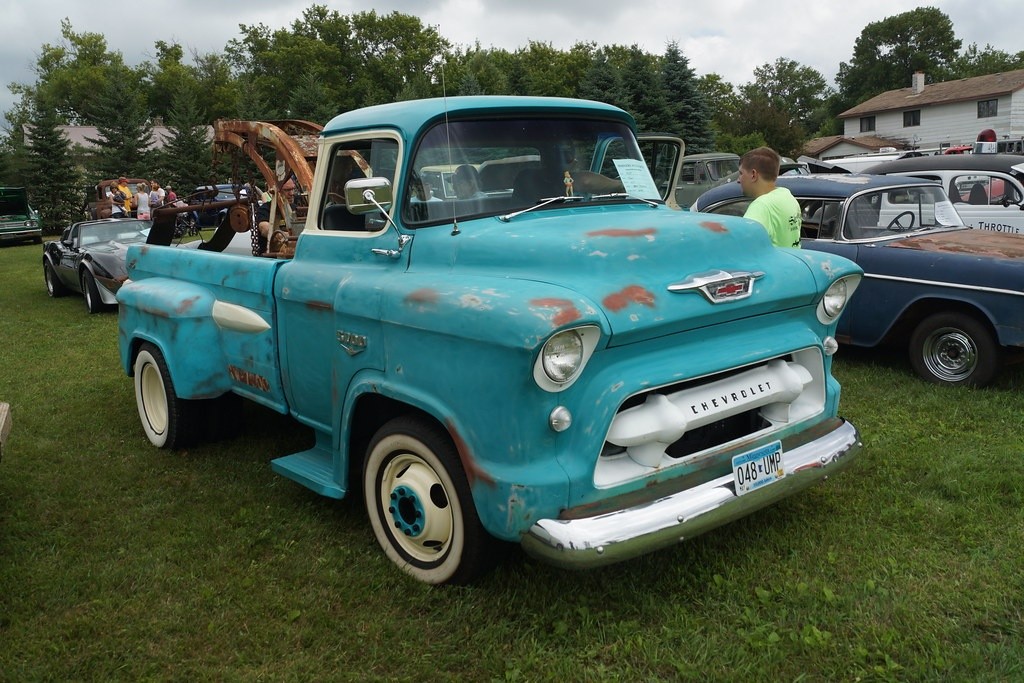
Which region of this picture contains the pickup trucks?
[116,94,868,586]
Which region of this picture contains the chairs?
[949,185,963,204]
[510,143,584,209]
[968,183,987,205]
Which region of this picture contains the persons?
[118,178,132,218]
[109,182,127,217]
[163,186,176,207]
[132,183,150,220]
[148,186,160,201]
[737,147,802,250]
[150,179,165,206]
[409,173,443,201]
[563,172,574,197]
[452,165,489,199]
[257,178,294,251]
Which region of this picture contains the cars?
[667,149,1024,241]
[187,184,264,228]
[0,186,43,246]
[684,173,1024,388]
[41,217,159,313]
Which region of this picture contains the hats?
[442,165,480,183]
[164,186,171,189]
[119,177,130,183]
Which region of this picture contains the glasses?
[111,186,117,189]
